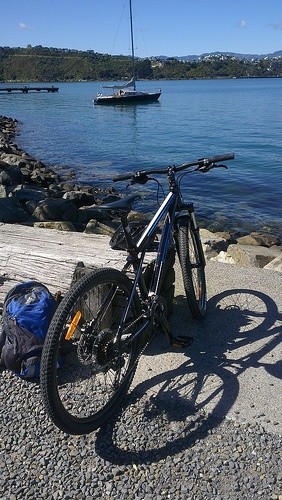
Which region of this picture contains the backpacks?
[0,281,58,379]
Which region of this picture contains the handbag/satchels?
[142,254,175,315]
[109,218,153,251]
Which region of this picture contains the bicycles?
[39,153,235,436]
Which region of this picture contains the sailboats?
[92,0,162,105]
